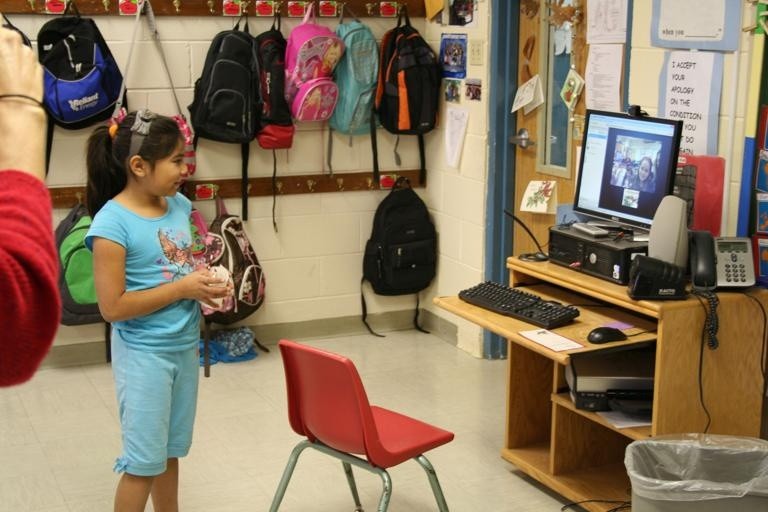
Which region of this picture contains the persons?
[626,156,656,193]
[84,108,234,512]
[1,25,63,389]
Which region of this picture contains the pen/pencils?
[612,231,625,242]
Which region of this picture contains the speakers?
[648,194,689,269]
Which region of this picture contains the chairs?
[268,338,454,512]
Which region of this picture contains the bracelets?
[0,94,43,107]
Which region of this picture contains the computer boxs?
[548,222,649,286]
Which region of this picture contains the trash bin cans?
[623,439,768,512]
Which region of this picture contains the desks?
[432,253,767,512]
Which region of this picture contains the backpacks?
[209,214,265,325]
[49,204,106,326]
[190,4,438,149]
[190,209,235,316]
[363,176,435,296]
[37,15,128,129]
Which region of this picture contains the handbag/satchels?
[109,111,196,179]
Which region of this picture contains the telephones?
[687,230,757,293]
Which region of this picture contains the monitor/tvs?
[572,109,684,242]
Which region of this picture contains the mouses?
[587,327,627,344]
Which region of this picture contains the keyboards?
[458,279,580,330]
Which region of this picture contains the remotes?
[572,222,609,238]
